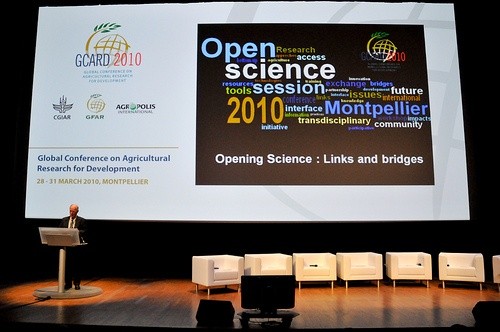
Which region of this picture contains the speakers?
[196,299,234,326]
[472,301,500,332]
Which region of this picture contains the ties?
[70,220,73,228]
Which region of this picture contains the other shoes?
[75,285,80,290]
[64,283,72,290]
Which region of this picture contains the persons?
[59,204,88,290]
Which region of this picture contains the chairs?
[244,253,293,275]
[386,252,432,288]
[439,252,485,290]
[336,252,383,288]
[293,253,337,288]
[492,255,500,292]
[191,255,245,295]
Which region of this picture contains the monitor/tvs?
[241,274,296,315]
